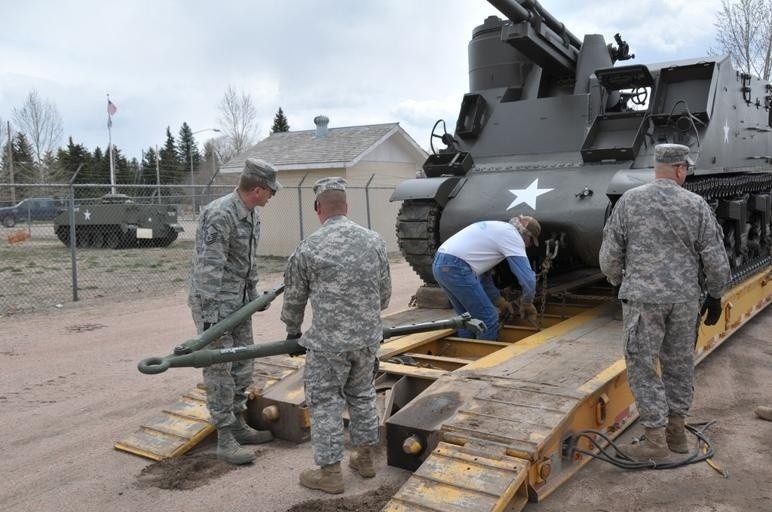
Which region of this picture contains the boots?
[350,446,375,478]
[617,426,669,457]
[231,412,274,445]
[640,415,688,454]
[300,461,345,494]
[217,426,256,464]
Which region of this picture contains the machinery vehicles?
[54,192,184,249]
[388,0,771,304]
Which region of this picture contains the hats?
[654,144,695,165]
[520,216,542,247]
[312,176,349,211]
[242,158,282,191]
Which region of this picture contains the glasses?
[252,186,276,196]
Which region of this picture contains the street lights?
[189,127,221,223]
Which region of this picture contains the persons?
[187,156,279,467]
[599,144,724,459]
[433,214,539,341]
[282,175,390,495]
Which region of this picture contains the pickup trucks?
[0,197,67,227]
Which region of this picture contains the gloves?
[287,333,307,357]
[520,303,537,321]
[700,292,722,325]
[495,297,514,321]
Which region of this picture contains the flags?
[106,93,118,129]
